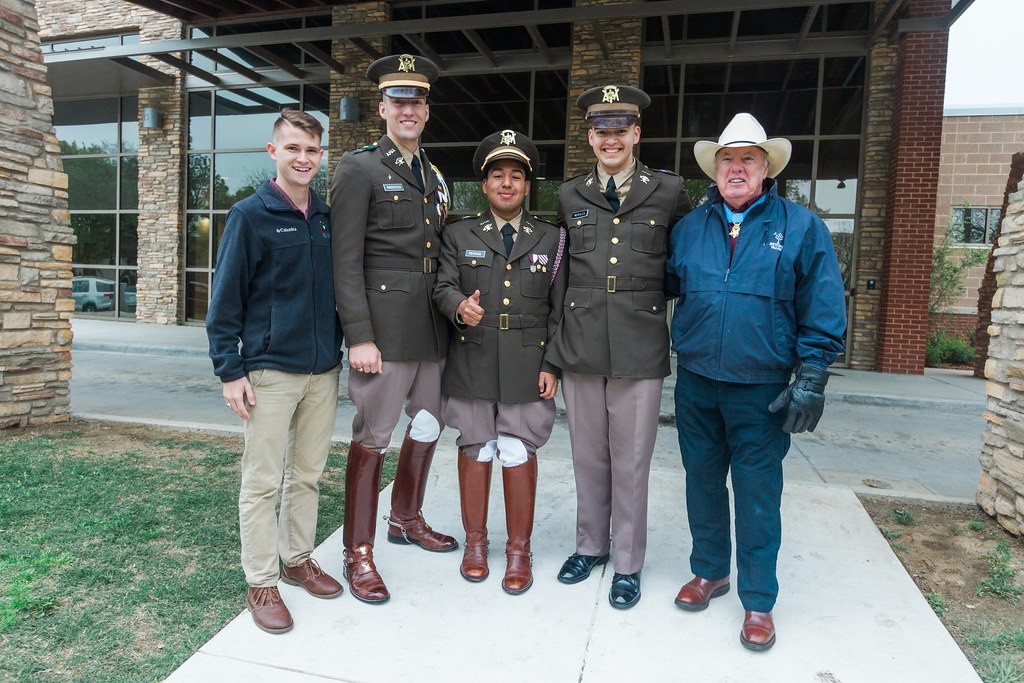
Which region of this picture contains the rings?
[226,403,232,409]
[357,368,363,372]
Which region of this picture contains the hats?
[366,54,440,98]
[694,113,793,184]
[575,84,651,129]
[473,130,540,181]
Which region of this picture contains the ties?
[410,154,426,192]
[502,223,517,258]
[604,177,620,214]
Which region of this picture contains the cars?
[72,276,116,312]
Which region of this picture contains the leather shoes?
[609,572,641,610]
[245,586,293,634]
[674,574,730,611]
[557,550,610,584]
[282,553,344,599]
[740,610,775,651]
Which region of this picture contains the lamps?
[339,97,359,123]
[143,107,161,130]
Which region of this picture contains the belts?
[568,274,664,292]
[364,256,437,274]
[480,312,548,329]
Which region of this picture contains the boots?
[343,440,391,604]
[502,454,537,594]
[388,429,458,552]
[459,446,493,583]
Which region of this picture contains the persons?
[329,53,459,605]
[664,111,847,653]
[431,129,562,595]
[557,83,694,609]
[206,108,345,635]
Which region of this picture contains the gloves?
[769,361,830,435]
